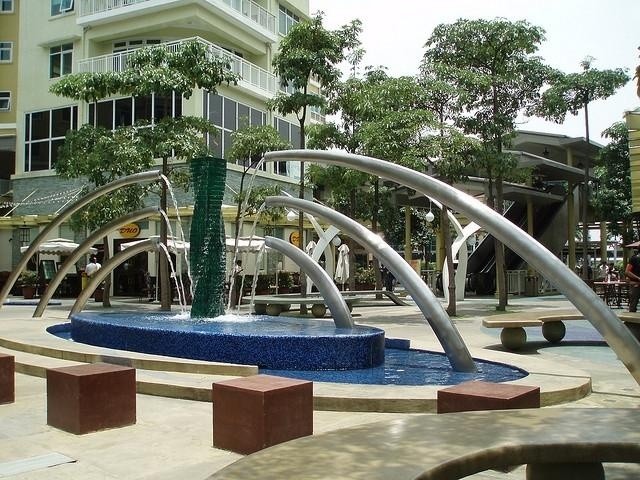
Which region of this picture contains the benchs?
[203,406,640,480]
[482,308,640,349]
[594,280,627,308]
[242,289,408,317]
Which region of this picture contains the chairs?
[137,268,154,302]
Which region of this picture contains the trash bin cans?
[524,275,538,297]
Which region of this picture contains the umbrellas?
[334,244,349,291]
[168,234,279,254]
[303,240,317,293]
[120,232,181,302]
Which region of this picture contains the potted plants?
[18,271,40,299]
[95,281,106,300]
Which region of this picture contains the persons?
[94,257,101,270]
[625,250,640,312]
[85,258,97,283]
[236,260,244,290]
[598,260,609,278]
[607,262,620,279]
